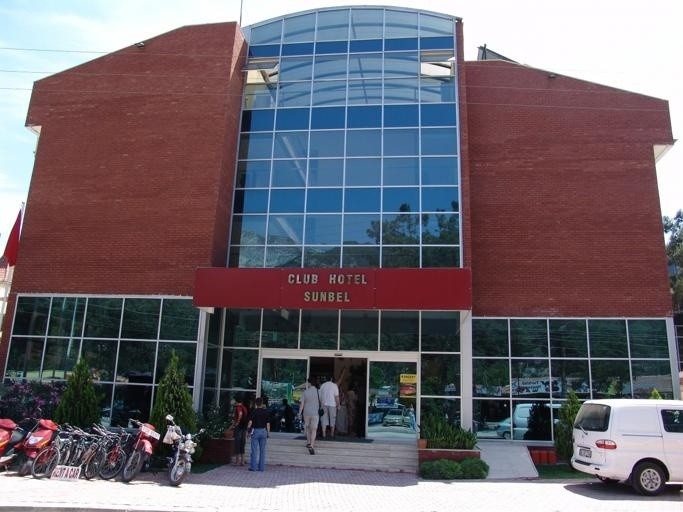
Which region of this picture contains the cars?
[99,408,140,427]
[368,409,410,426]
[380,386,391,395]
[496,404,561,440]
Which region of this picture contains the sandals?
[306,444,315,455]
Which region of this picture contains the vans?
[571,399,683,496]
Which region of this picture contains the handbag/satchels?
[318,409,324,417]
[238,406,247,427]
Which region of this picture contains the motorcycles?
[122,415,206,486]
[295,420,303,432]
[0,419,61,476]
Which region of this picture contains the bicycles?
[31,423,135,479]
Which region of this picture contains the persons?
[282,399,295,421]
[346,384,359,438]
[335,384,349,436]
[298,376,320,455]
[319,374,341,441]
[247,397,271,471]
[227,394,248,466]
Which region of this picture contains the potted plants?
[415,411,482,464]
[194,392,236,465]
